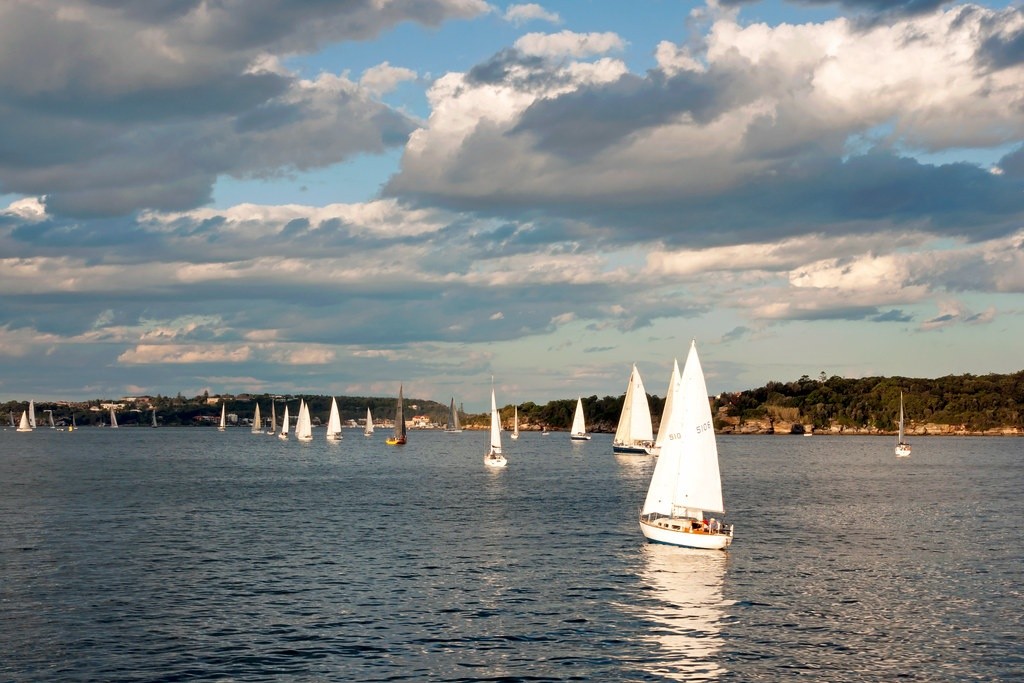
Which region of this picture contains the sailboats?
[278,405,289,438]
[386,384,407,445]
[639,339,734,550]
[612,363,655,453]
[110,408,119,428]
[16,410,33,432]
[267,401,276,435]
[251,403,264,434]
[570,396,592,441]
[217,403,226,430]
[643,357,682,456]
[326,396,344,441]
[29,399,37,428]
[483,389,508,467]
[511,404,519,439]
[895,390,911,456]
[295,399,313,442]
[444,397,463,433]
[364,407,375,436]
